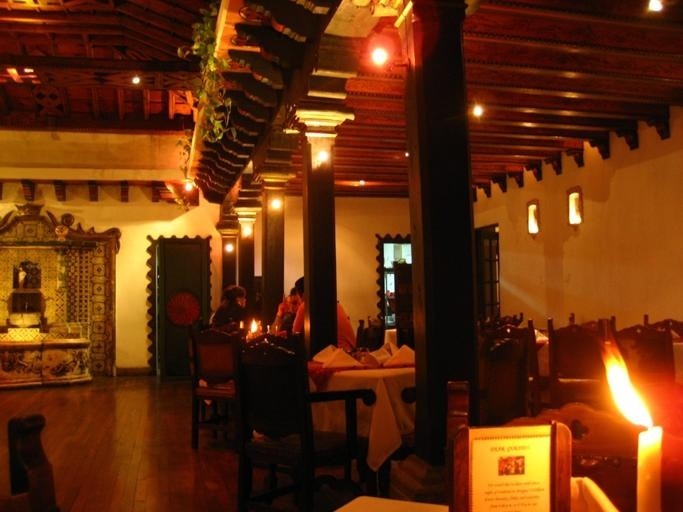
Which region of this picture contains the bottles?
[265,325,271,344]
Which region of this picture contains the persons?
[292,275,356,353]
[211,286,248,331]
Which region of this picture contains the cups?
[352,347,370,365]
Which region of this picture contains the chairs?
[189,312,683,511]
